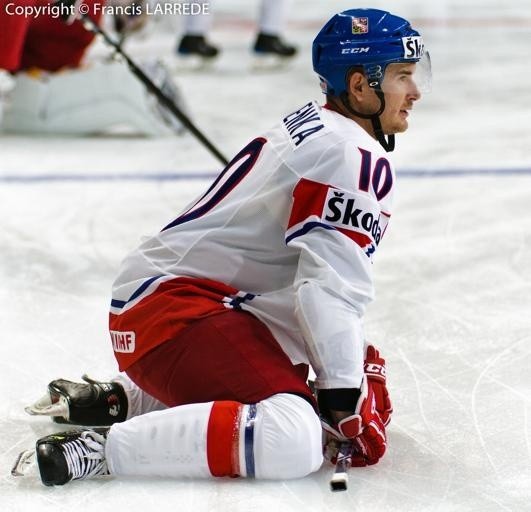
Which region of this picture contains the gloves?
[317,344,394,470]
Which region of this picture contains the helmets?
[312,8,426,99]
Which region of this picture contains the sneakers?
[36,430,113,488]
[48,375,129,428]
[253,33,295,55]
[178,32,217,56]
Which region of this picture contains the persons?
[35,7,426,488]
[0,0,297,139]
